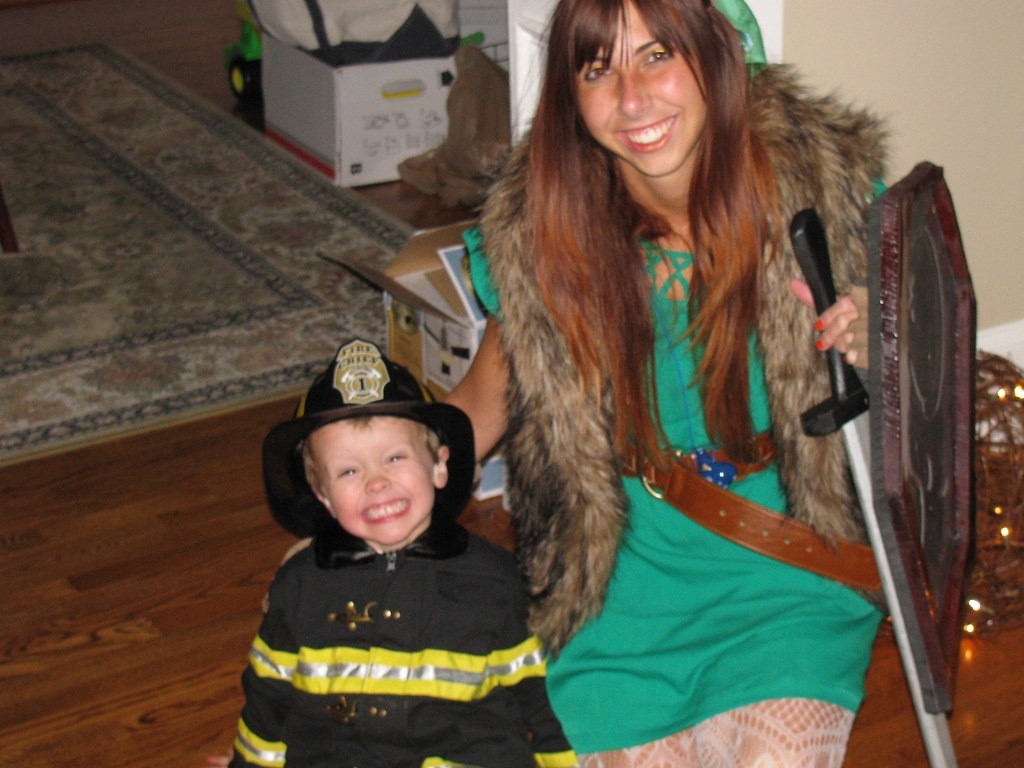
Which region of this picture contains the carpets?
[0,38,419,472]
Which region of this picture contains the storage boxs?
[260,34,457,191]
[317,218,491,396]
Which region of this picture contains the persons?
[446,0,888,768]
[227,340,575,768]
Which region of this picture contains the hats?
[263,337,480,539]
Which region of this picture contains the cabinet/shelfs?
[507,1,785,154]
